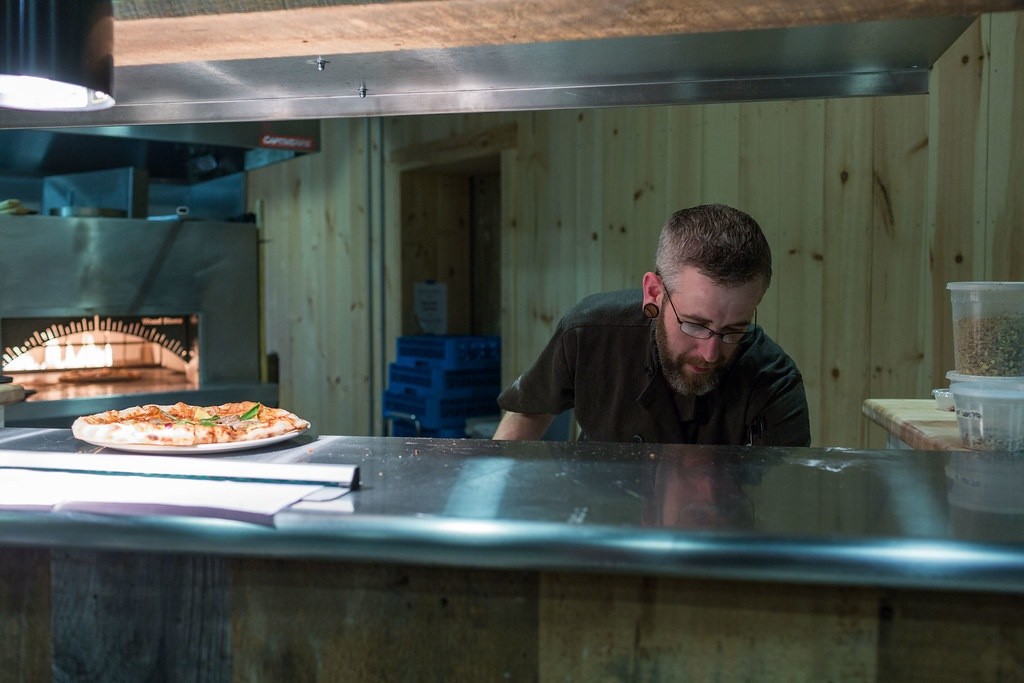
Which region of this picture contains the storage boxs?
[382,332,503,440]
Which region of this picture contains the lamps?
[0,0,117,114]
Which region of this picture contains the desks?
[859,398,974,454]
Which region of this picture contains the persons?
[477,441,799,534]
[489,203,813,446]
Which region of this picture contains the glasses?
[657,271,759,344]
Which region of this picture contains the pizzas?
[71,401,310,445]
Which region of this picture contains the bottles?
[947,278,1024,378]
[947,381,1024,457]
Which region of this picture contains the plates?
[73,418,313,452]
